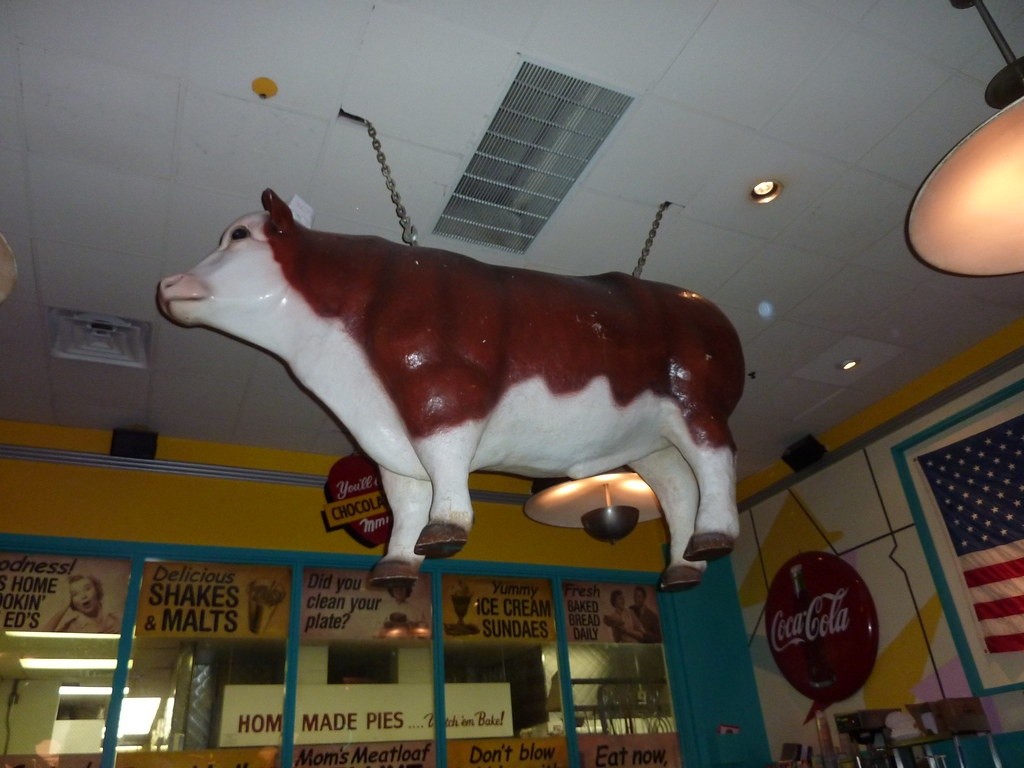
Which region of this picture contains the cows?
[154,186,744,595]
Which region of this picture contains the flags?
[914,415,1023,657]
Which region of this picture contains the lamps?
[521,460,665,546]
[903,0,1024,278]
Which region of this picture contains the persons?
[42,575,120,634]
[379,586,426,623]
[604,587,661,644]
